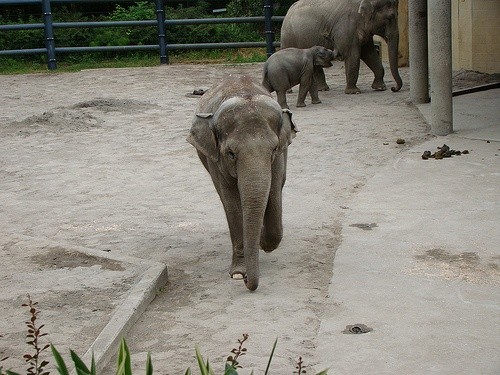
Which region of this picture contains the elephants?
[261,32,339,110]
[185,72,301,292]
[280,0,403,95]
